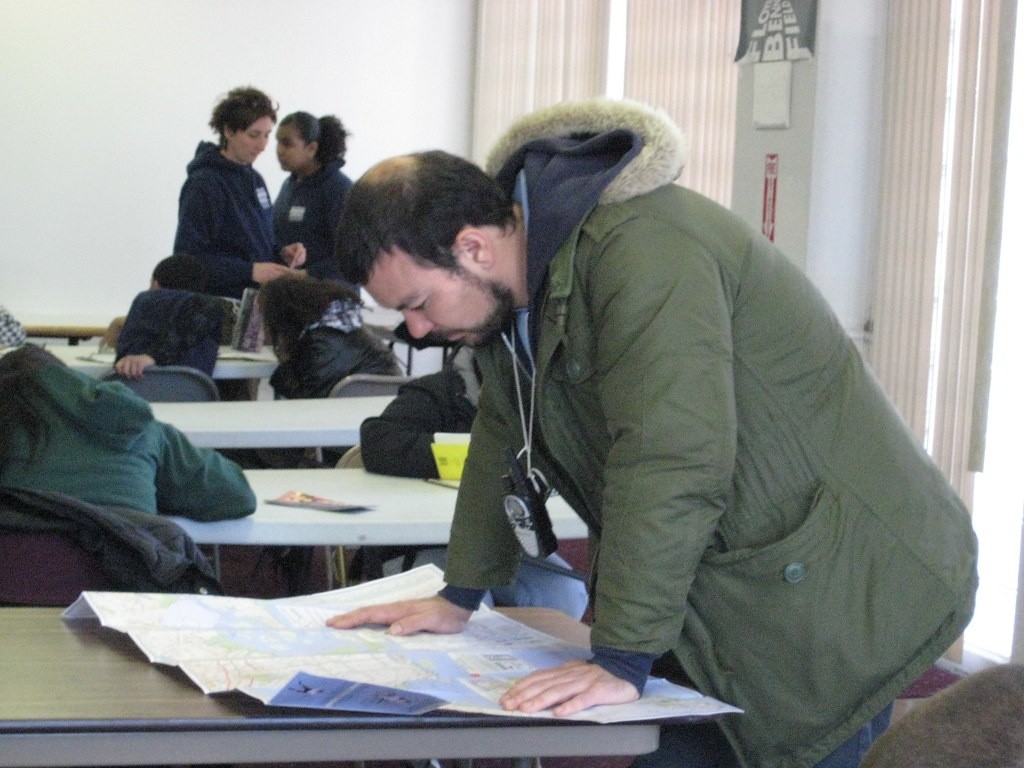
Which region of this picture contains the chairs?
[303,375,423,465]
[0,486,222,608]
[98,366,220,402]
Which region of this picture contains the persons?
[325,98,979,768]
[117,87,482,586]
[0,344,257,522]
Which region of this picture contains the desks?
[41,344,279,380]
[158,469,591,599]
[150,394,404,450]
[360,307,448,376]
[0,607,659,768]
